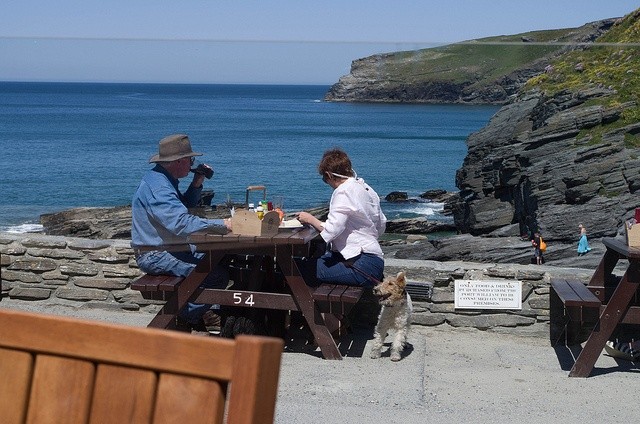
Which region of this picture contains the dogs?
[369,271,414,363]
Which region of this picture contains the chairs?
[0,310,282,423]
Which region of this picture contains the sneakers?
[201,310,221,331]
[177,316,210,336]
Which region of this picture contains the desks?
[559,235,640,377]
[147,224,345,359]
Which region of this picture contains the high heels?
[303,325,348,353]
[340,315,351,335]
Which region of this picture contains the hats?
[149,134,203,163]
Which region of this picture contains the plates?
[278,225,304,228]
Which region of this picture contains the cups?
[190,158,214,179]
[273,196,283,212]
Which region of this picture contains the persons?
[532,230,545,266]
[291,149,390,348]
[577,223,590,258]
[129,133,235,335]
[605,340,640,362]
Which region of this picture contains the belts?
[133,246,167,253]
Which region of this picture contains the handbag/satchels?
[539,236,547,251]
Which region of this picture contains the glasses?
[322,174,327,182]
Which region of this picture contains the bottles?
[248,203,254,212]
[256,207,264,220]
[267,201,273,211]
[261,200,269,211]
[258,201,262,207]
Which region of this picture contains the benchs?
[550,277,600,309]
[313,283,363,306]
[132,272,182,299]
